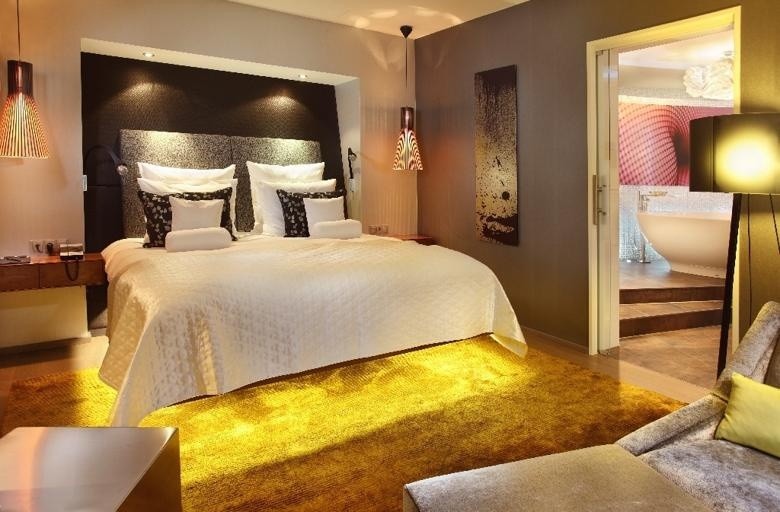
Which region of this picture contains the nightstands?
[0,254,107,352]
[393,235,434,244]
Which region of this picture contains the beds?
[93,129,533,434]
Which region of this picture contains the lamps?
[679,56,744,103]
[393,25,423,172]
[1,1,57,159]
[684,115,779,399]
[346,145,359,198]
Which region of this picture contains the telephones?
[59,242,84,259]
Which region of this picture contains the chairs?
[394,294,780,510]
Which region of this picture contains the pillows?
[131,159,365,254]
[715,372,775,460]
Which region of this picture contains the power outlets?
[44,240,57,253]
[31,240,43,253]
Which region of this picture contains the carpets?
[0,325,701,511]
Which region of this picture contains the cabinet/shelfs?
[0,423,185,511]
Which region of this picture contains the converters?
[47,243,53,253]
